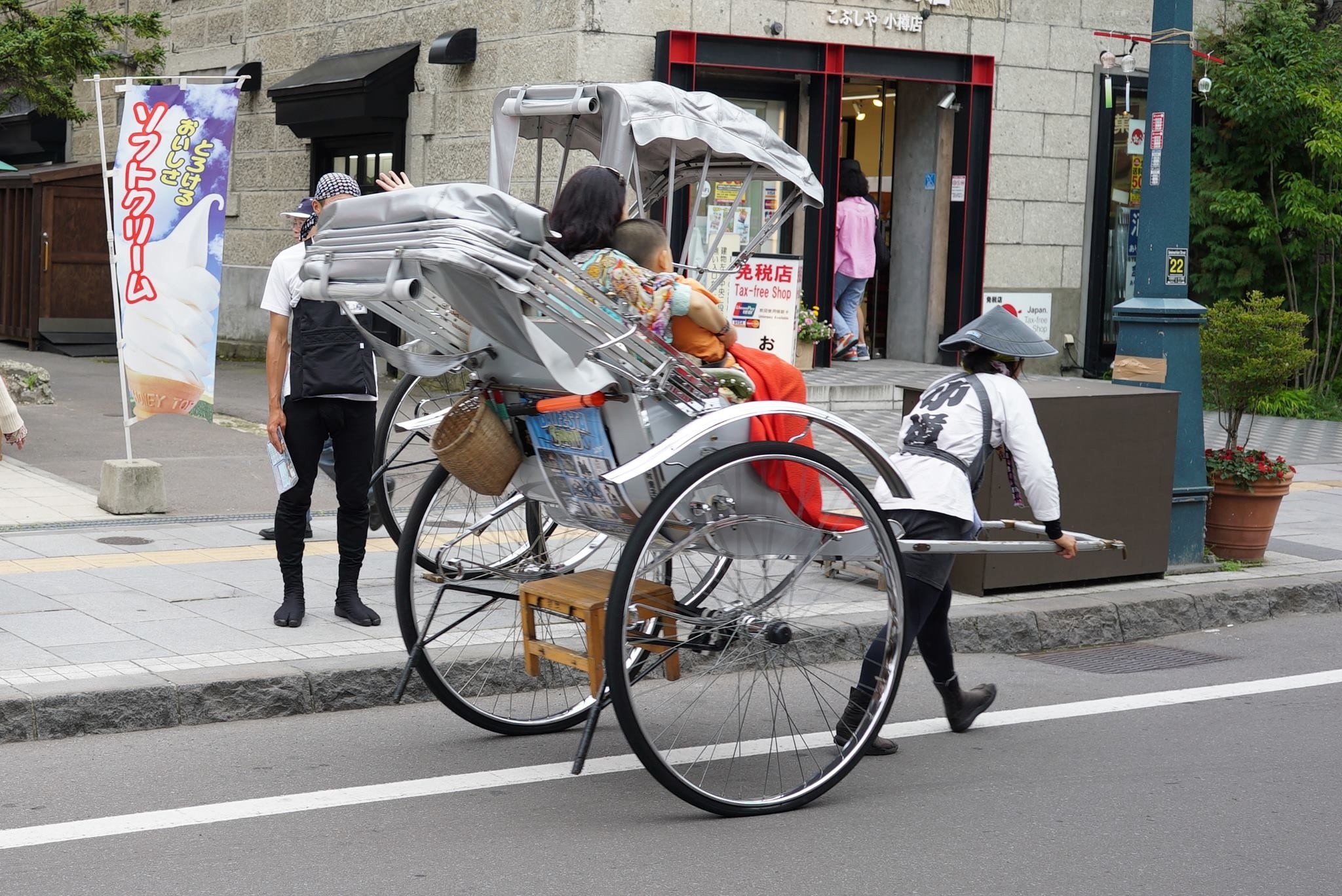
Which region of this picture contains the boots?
[273,562,305,627]
[333,563,381,627]
[933,673,996,733]
[834,686,898,755]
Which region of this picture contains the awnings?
[267,45,419,138]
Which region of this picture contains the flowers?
[797,300,836,345]
[1200,289,1317,493]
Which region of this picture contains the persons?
[260,197,396,539]
[0,376,27,449]
[831,158,879,362]
[258,170,414,627]
[834,305,1078,756]
[529,164,863,531]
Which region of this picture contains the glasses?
[585,164,627,185]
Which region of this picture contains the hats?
[279,197,316,218]
[939,305,1058,358]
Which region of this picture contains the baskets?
[429,393,523,496]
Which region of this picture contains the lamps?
[428,28,477,65]
[852,100,865,121]
[222,62,262,91]
[872,88,883,107]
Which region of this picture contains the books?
[266,426,299,494]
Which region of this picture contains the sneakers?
[832,347,858,361]
[832,333,859,358]
[857,345,869,360]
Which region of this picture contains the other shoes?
[369,477,394,531]
[259,524,313,539]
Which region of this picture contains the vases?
[796,341,814,370]
[1205,472,1294,560]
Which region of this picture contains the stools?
[519,569,680,699]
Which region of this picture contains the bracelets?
[715,320,730,337]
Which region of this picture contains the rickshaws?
[296,79,1128,818]
